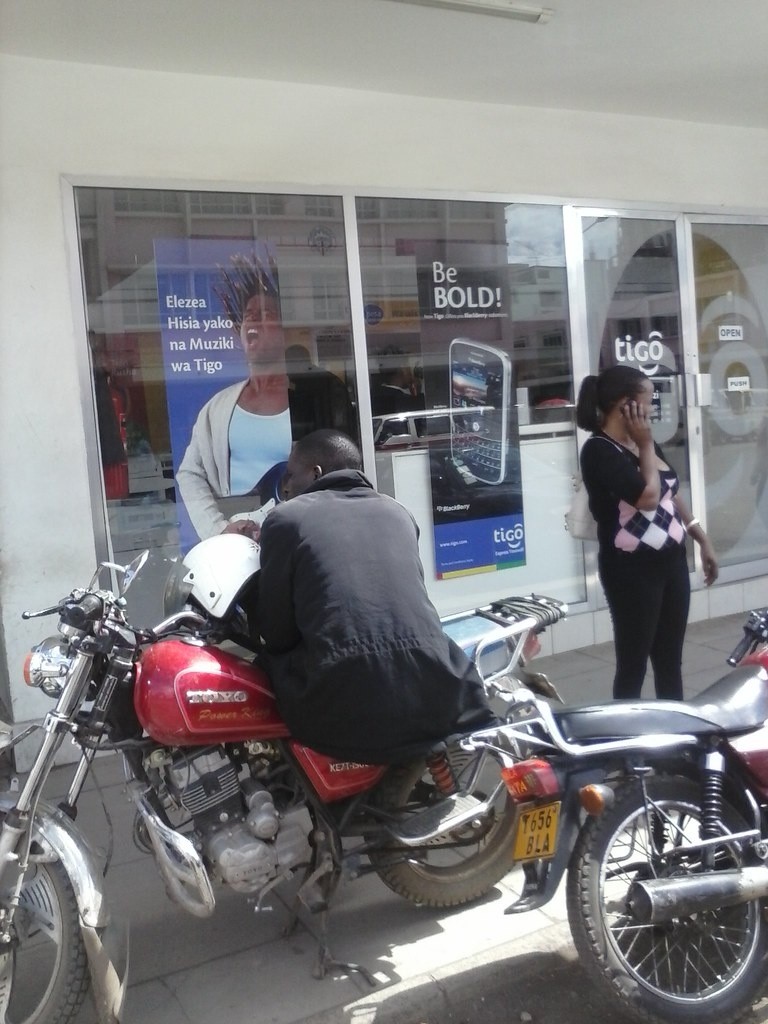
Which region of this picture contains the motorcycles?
[0,540,569,1021]
[459,600,767,1020]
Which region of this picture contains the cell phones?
[620,400,647,420]
[449,338,512,486]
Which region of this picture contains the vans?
[373,405,496,473]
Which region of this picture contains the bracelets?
[685,517,699,530]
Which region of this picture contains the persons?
[282,344,355,448]
[213,429,491,764]
[381,345,426,441]
[574,363,719,701]
[167,245,294,543]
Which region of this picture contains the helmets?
[164,531,263,629]
